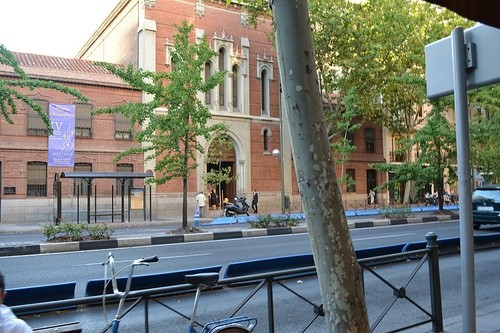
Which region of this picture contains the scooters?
[223,195,252,217]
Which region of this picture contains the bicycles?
[89,251,259,333]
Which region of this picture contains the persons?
[367,189,375,206]
[252,189,258,214]
[209,189,218,210]
[0,273,33,333]
[425,190,453,206]
[196,192,206,217]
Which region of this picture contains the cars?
[470,183,500,229]
